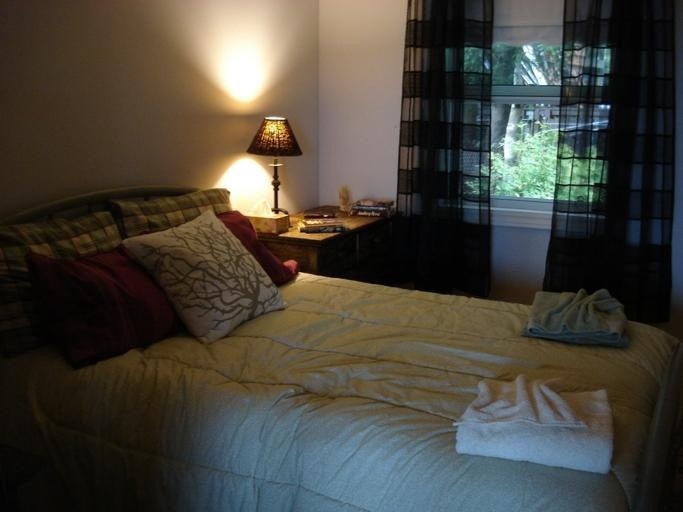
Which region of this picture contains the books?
[349,198,394,217]
[297,218,345,233]
[303,206,340,219]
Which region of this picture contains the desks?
[247,201,405,277]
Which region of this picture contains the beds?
[1,182,683,512]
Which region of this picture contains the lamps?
[244,113,305,227]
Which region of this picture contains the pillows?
[109,186,235,239]
[16,248,175,369]
[120,208,289,346]
[144,210,295,304]
[0,211,124,357]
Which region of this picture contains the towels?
[452,373,614,474]
[520,288,631,349]
